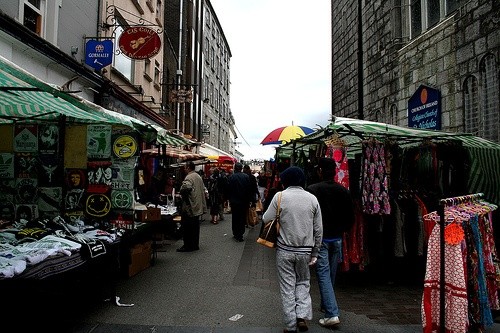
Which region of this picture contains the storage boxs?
[129,241,151,277]
[133,208,161,222]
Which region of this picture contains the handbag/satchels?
[256,191,281,248]
[256,197,263,212]
[248,207,259,227]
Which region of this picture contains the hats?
[313,157,336,169]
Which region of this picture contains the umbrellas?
[259,119,315,153]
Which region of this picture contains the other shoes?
[239,238,245,242]
[217,218,225,221]
[318,316,340,327]
[297,317,308,332]
[210,219,218,224]
[177,246,190,252]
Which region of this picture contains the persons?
[134,154,260,253]
[307,156,356,327]
[261,157,324,333]
[69,170,81,188]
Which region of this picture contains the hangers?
[396,189,420,201]
[325,131,348,148]
[422,193,497,222]
[295,144,309,164]
[364,130,383,143]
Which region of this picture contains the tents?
[269,115,500,203]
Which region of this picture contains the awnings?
[0,58,241,168]
